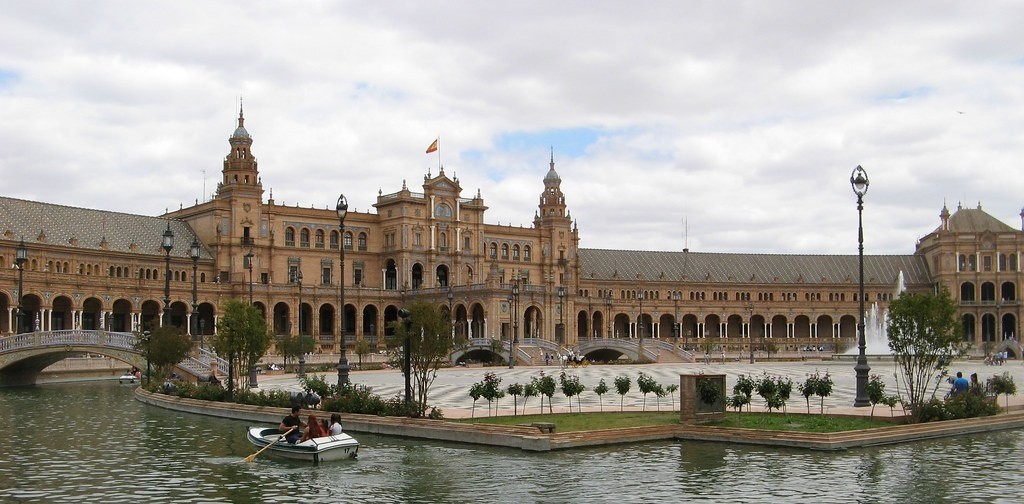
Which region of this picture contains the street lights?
[161,220,174,327]
[558,286,565,354]
[748,304,754,363]
[401,290,405,306]
[447,287,453,338]
[636,288,645,359]
[336,194,349,392]
[849,162,873,406]
[512,282,519,348]
[16,234,27,345]
[248,248,258,388]
[190,233,199,340]
[296,271,307,378]
[605,295,612,337]
[508,297,513,368]
[674,293,681,344]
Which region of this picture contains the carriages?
[556,350,592,370]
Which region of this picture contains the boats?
[247,426,361,463]
[119,375,148,383]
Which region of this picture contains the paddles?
[244,425,299,461]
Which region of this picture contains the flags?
[426,139,437,154]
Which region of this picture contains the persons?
[301,414,322,442]
[124,366,140,378]
[568,349,573,357]
[803,345,823,351]
[550,354,554,363]
[268,363,277,370]
[983,349,1009,366]
[951,372,969,397]
[318,419,329,436]
[544,353,550,366]
[721,353,725,364]
[329,413,343,436]
[969,373,983,393]
[704,352,710,365]
[278,405,305,444]
